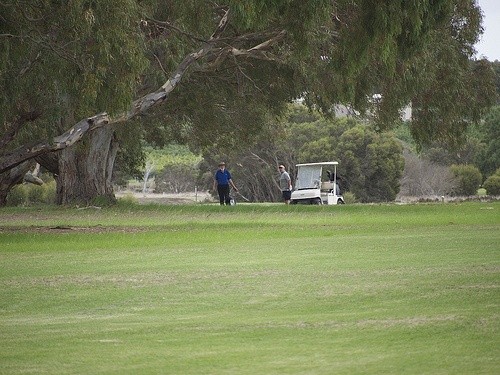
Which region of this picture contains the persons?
[213,162,238,206]
[278,164,293,206]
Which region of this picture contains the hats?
[217,161,226,166]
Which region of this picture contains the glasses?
[279,167,283,168]
